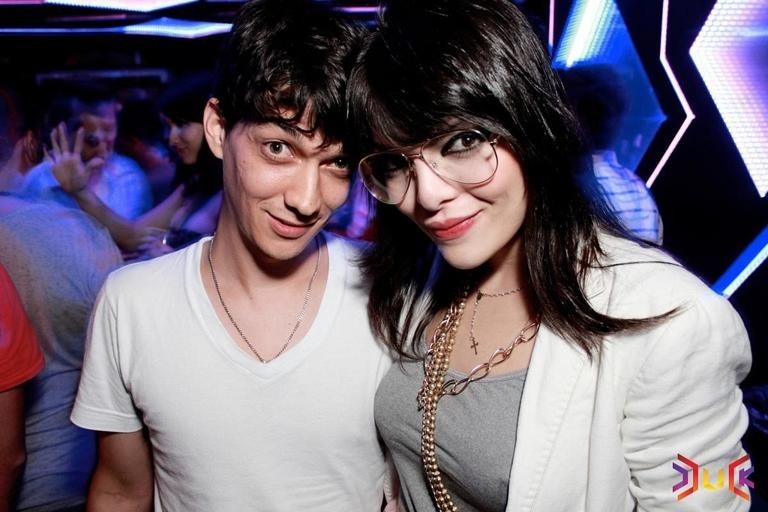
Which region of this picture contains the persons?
[546,57,670,253]
[1,59,226,510]
[342,0,756,512]
[66,0,420,510]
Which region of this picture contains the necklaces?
[208,227,325,365]
[417,281,545,414]
[457,267,534,356]
[417,270,469,512]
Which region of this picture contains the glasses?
[354,125,502,207]
